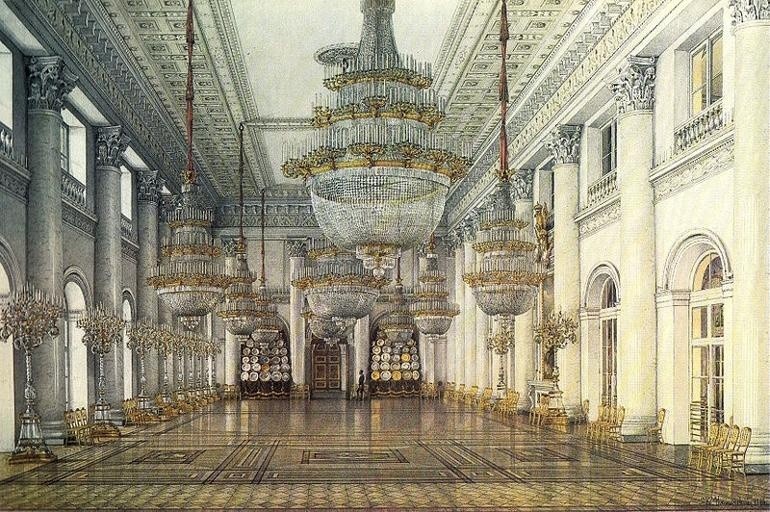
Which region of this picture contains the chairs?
[62,383,248,447]
[288,382,313,400]
[683,422,757,482]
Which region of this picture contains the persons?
[357,370,364,401]
